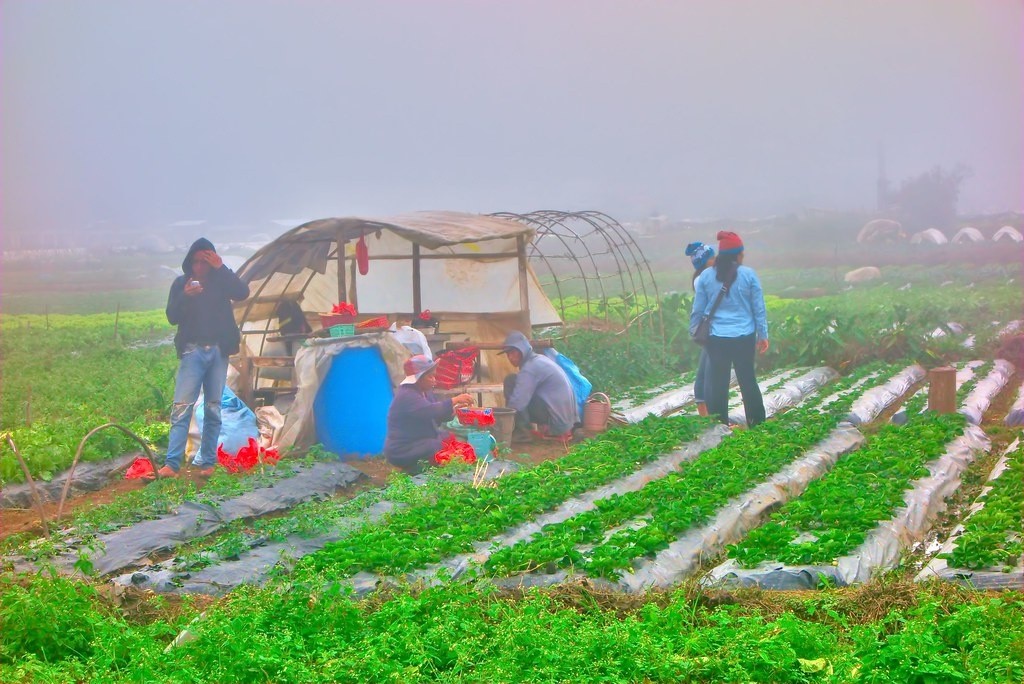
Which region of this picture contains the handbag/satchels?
[435,347,481,389]
[693,318,711,344]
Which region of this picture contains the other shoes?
[513,429,532,443]
[159,466,177,476]
[201,467,216,477]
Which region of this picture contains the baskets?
[328,323,355,337]
[583,392,611,431]
[318,311,354,329]
[357,316,389,328]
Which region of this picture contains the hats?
[685,242,715,269]
[400,355,441,387]
[717,230,744,253]
[495,346,516,355]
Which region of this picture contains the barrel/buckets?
[486,406,517,447]
[313,345,395,461]
[447,421,478,441]
[583,392,611,431]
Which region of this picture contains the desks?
[432,382,507,395]
[444,339,553,406]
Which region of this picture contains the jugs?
[467,431,497,463]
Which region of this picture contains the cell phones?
[190,281,199,286]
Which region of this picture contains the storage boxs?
[328,323,354,337]
[318,311,353,328]
[414,324,435,335]
[354,314,389,329]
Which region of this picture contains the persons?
[689,231,768,429]
[684,242,716,415]
[385,354,475,475]
[495,329,574,445]
[156,238,250,477]
